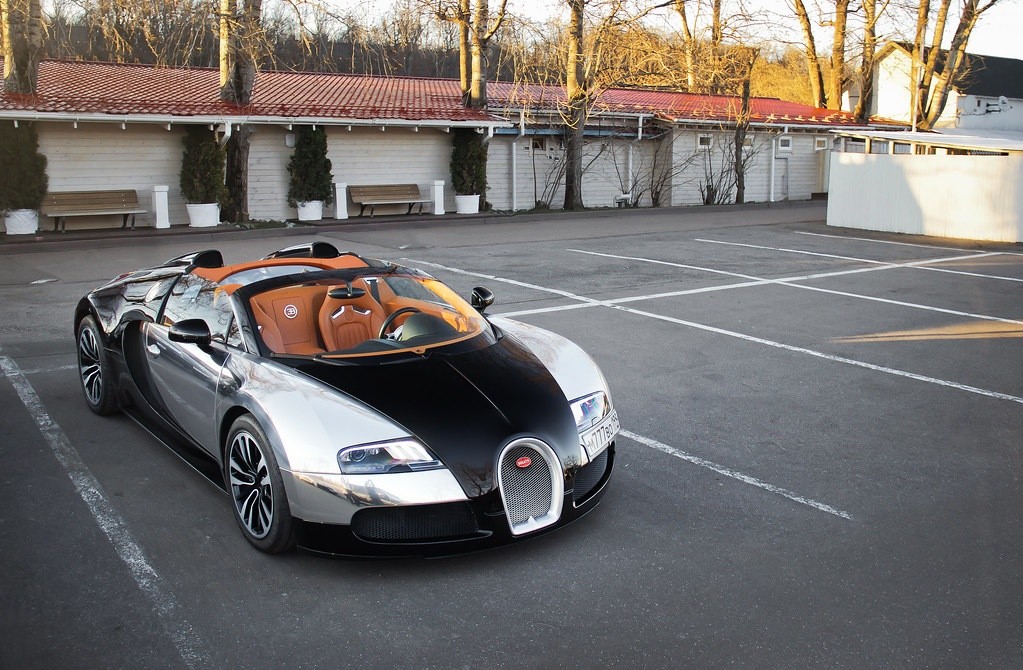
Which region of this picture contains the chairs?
[318,278,390,352]
[213,284,285,354]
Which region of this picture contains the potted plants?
[285,125,334,220]
[0,120,49,234]
[179,125,233,228]
[449,129,488,214]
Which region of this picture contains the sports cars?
[72,240,624,560]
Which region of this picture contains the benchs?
[40,189,147,233]
[346,184,432,217]
